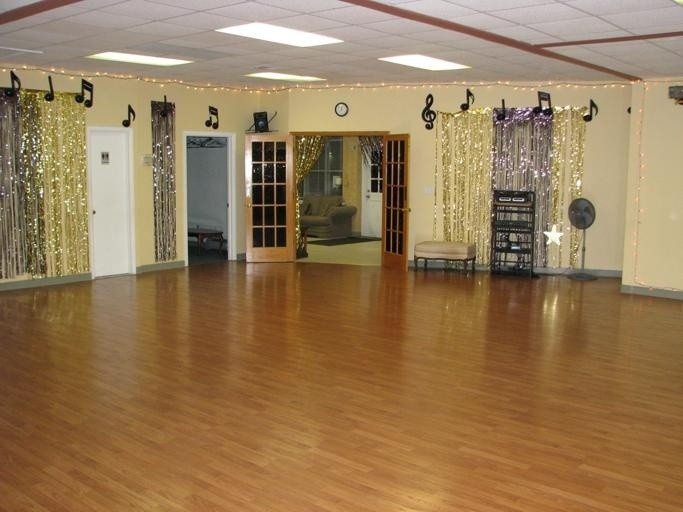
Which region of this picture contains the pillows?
[299,203,310,215]
[319,199,341,217]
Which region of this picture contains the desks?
[188,228,224,257]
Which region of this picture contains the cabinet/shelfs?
[491,190,536,278]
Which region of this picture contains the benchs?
[414,241,476,275]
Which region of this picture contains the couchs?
[299,195,357,238]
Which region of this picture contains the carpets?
[306,237,381,246]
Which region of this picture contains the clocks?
[335,103,349,116]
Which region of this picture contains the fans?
[567,198,597,281]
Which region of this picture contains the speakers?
[253,112,269,132]
